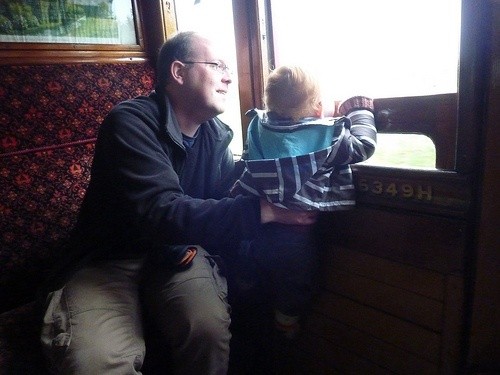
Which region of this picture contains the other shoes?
[267,307,304,338]
[231,269,258,293]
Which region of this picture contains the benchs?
[0,63,164,375]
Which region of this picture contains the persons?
[231,66,379,340]
[48,25,323,374]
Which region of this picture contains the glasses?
[181,59,234,73]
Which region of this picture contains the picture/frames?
[1,0,152,64]
